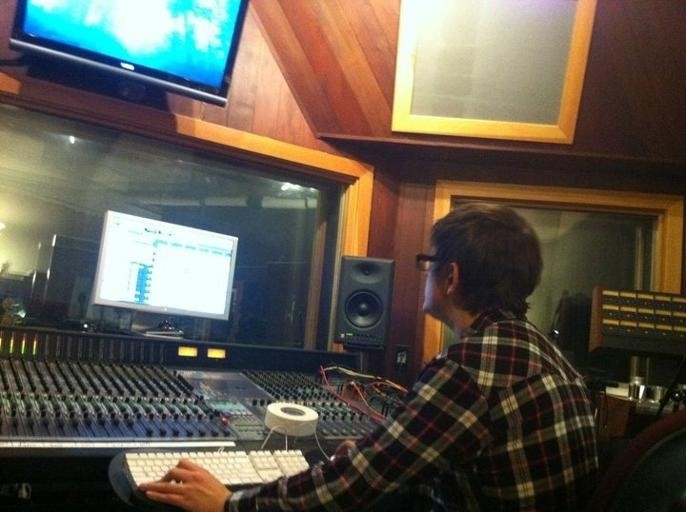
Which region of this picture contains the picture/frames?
[392,0,598,146]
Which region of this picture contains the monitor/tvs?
[89,210,240,323]
[8,0,247,109]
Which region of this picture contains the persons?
[138,202,599,512]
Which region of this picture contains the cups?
[629,354,649,388]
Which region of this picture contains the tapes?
[264,402,318,436]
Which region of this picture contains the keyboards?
[120,447,313,495]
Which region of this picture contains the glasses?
[414,253,440,272]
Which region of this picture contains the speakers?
[333,254,395,355]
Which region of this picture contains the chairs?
[591,413,686,512]
